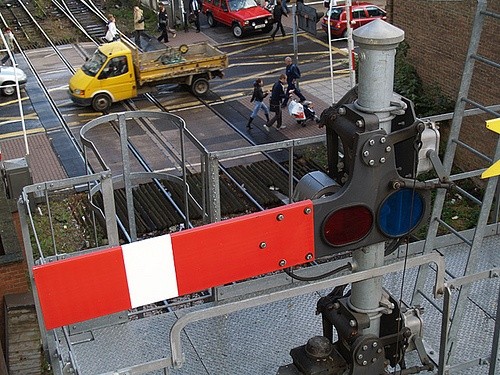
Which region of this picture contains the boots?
[246,116,254,130]
[264,114,270,122]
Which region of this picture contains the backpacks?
[290,64,302,80]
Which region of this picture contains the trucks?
[66,34,228,114]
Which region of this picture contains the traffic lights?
[311,179,432,258]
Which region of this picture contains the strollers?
[290,89,321,128]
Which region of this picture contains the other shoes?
[1,60,5,66]
[196,29,200,33]
[276,125,287,131]
[263,125,270,133]
[298,99,306,103]
[270,35,276,40]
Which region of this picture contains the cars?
[202,0,274,39]
[0,66,28,97]
[321,2,387,42]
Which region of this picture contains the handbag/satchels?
[288,100,307,120]
[136,16,145,24]
[270,104,279,112]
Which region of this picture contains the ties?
[194,0,198,11]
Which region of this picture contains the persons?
[158,3,176,38]
[106,14,113,29]
[246,78,270,130]
[157,6,169,43]
[105,18,117,42]
[2,27,18,67]
[264,73,294,131]
[281,57,306,108]
[279,0,290,14]
[189,0,202,33]
[133,4,153,47]
[111,57,127,76]
[271,0,289,41]
